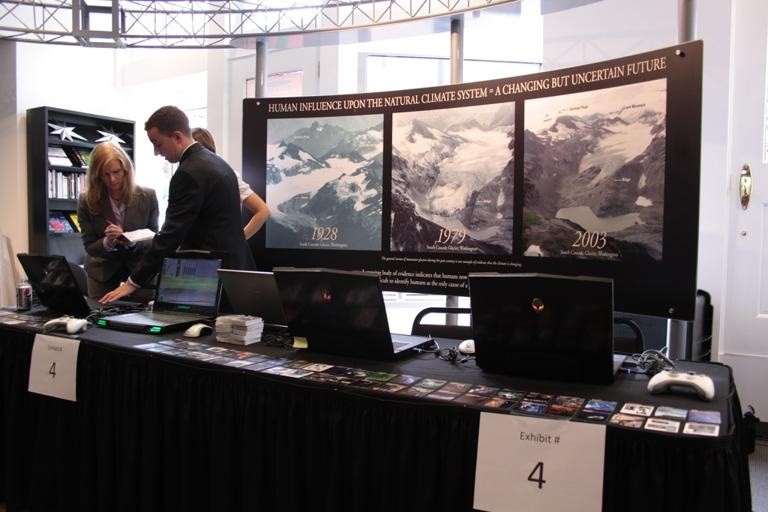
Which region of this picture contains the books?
[215,313,264,348]
[68,212,82,233]
[48,211,73,234]
[49,145,91,202]
[115,227,158,243]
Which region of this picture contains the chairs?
[410,303,479,340]
[607,314,648,358]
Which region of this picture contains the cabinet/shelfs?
[23,104,135,268]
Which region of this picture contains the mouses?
[459,339,475,354]
[182,323,213,337]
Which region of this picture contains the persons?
[187,127,272,240]
[98,105,256,306]
[76,141,159,306]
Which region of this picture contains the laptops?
[18,254,144,317]
[468,272,628,386]
[216,269,288,328]
[273,267,434,363]
[97,249,230,336]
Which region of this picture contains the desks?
[1,293,760,511]
